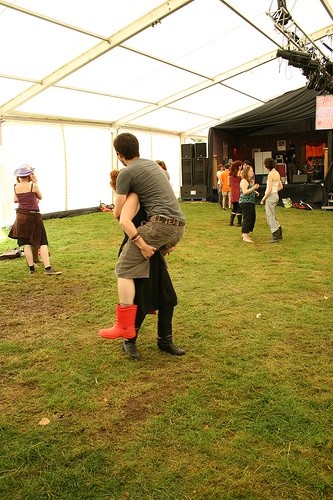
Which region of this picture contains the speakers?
[181,143,209,186]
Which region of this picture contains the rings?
[131,236,141,243]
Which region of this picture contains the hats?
[14,165,31,176]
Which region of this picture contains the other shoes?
[44,270,62,275]
[35,259,44,264]
[228,209,231,212]
[242,239,253,242]
[242,236,250,239]
[222,209,225,211]
[30,270,38,276]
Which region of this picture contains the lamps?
[276,39,333,96]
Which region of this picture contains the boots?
[123,329,142,360]
[278,226,282,239]
[237,215,242,227]
[157,334,178,355]
[99,304,137,339]
[229,214,236,226]
[269,229,279,242]
[149,310,156,314]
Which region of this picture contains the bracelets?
[131,231,140,240]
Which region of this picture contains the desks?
[292,174,311,183]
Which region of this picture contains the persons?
[97,132,186,340]
[110,167,187,360]
[260,158,283,243]
[216,164,233,212]
[285,144,297,183]
[8,164,63,276]
[238,166,259,243]
[228,160,255,227]
[155,160,170,180]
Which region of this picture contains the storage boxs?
[254,151,272,175]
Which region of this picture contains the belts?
[149,216,185,227]
[30,211,40,214]
